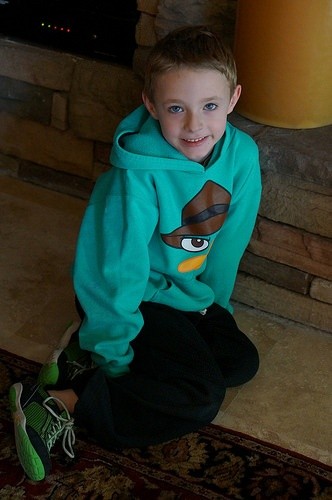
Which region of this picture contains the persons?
[7,25,263,482]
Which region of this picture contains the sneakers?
[8,380,76,481]
[38,319,95,389]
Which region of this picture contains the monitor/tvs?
[0,0,141,73]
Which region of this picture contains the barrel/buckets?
[229,0,332,134]
